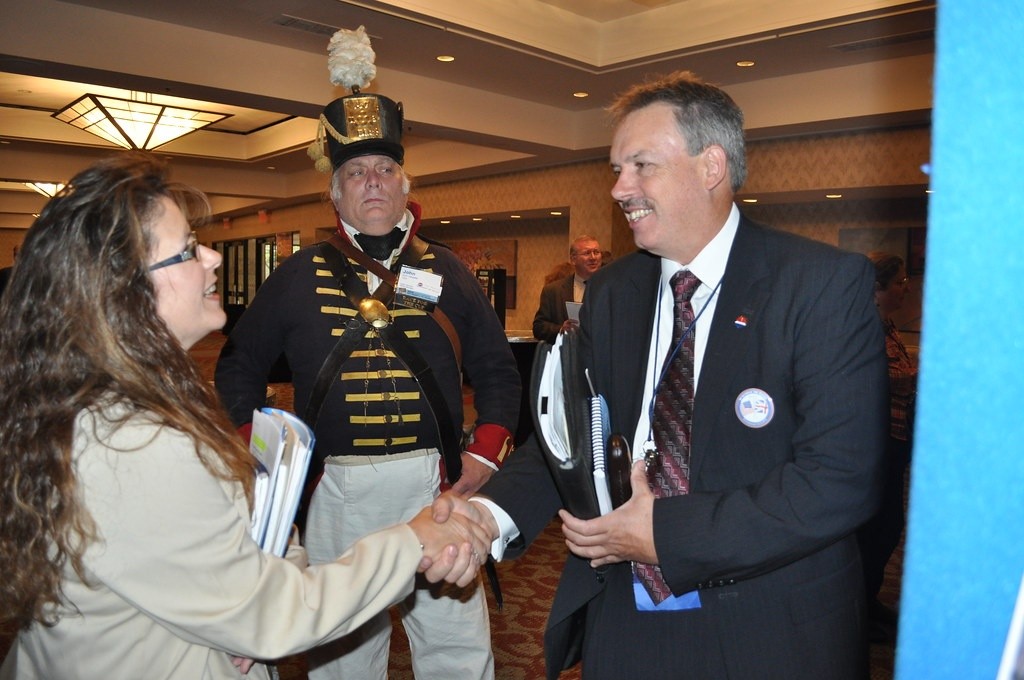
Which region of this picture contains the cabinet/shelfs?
[476,269,507,330]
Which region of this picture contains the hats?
[307,24,405,176]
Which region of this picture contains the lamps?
[50,89,235,153]
[21,182,77,200]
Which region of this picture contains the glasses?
[893,276,910,284]
[142,230,199,274]
[572,248,602,257]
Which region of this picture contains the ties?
[631,269,704,607]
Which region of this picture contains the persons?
[532,234,613,346]
[214,25,522,680]
[0,245,22,299]
[856,252,917,645]
[0,148,493,680]
[416,71,892,680]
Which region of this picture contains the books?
[240,406,317,560]
